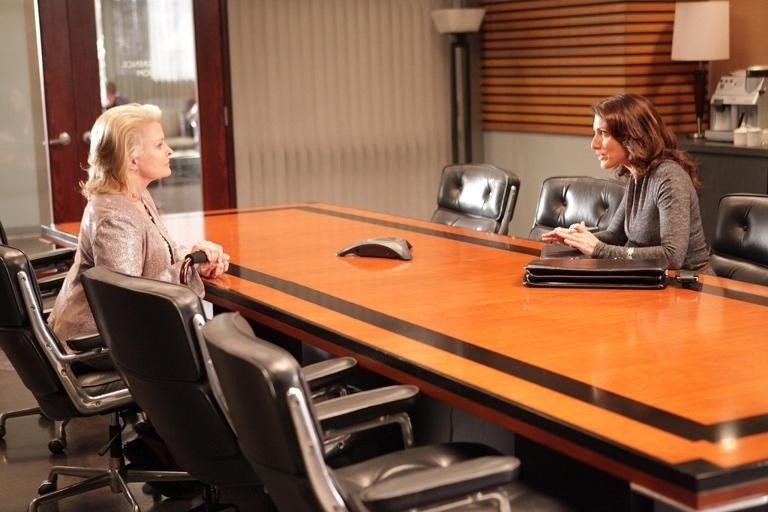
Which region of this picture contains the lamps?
[669,3,732,139]
[432,8,490,159]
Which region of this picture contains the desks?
[45,203,768,512]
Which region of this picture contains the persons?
[102,81,129,110]
[48,103,229,349]
[539,93,711,273]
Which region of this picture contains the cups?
[733,128,763,149]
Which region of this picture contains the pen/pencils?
[568,226,598,233]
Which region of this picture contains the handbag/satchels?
[522,256,697,290]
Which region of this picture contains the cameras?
[675,271,698,289]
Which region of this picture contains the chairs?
[526,174,626,245]
[429,162,522,236]
[196,310,522,510]
[0,217,144,512]
[83,264,420,511]
[708,191,768,285]
